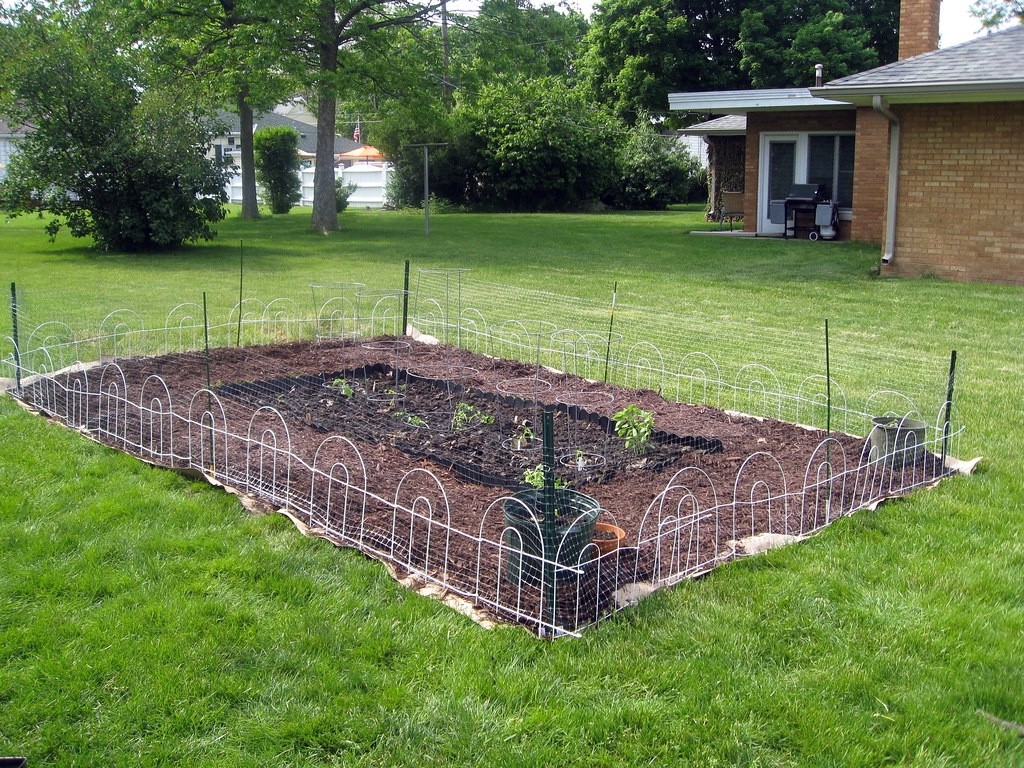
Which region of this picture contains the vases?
[592,522,626,559]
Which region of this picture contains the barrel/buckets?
[871,416,929,469]
[503,486,600,583]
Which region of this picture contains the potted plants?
[504,462,602,592]
[871,416,929,471]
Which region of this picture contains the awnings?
[339,145,386,165]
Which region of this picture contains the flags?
[354,120,359,143]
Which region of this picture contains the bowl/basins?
[591,522,626,560]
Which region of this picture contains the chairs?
[719,189,744,232]
